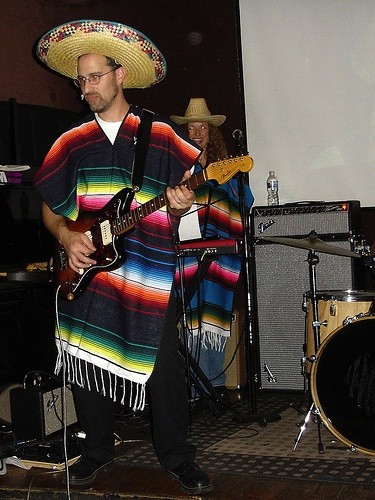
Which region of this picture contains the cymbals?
[259,235,361,257]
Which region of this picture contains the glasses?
[74,64,122,87]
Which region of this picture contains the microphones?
[233,128,243,152]
[80,94,85,101]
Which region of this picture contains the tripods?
[292,254,324,453]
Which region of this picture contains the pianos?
[171,239,240,418]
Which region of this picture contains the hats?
[170,98,226,126]
[36,20,167,88]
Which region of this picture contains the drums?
[302,289,375,375]
[309,317,375,455]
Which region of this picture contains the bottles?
[266,171,279,206]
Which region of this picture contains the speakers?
[11,379,79,438]
[251,200,361,393]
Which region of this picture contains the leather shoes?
[63,455,114,487]
[213,385,240,401]
[167,462,213,494]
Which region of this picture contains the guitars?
[48,152,253,301]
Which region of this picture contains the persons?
[171,97,254,408]
[36,19,213,494]
